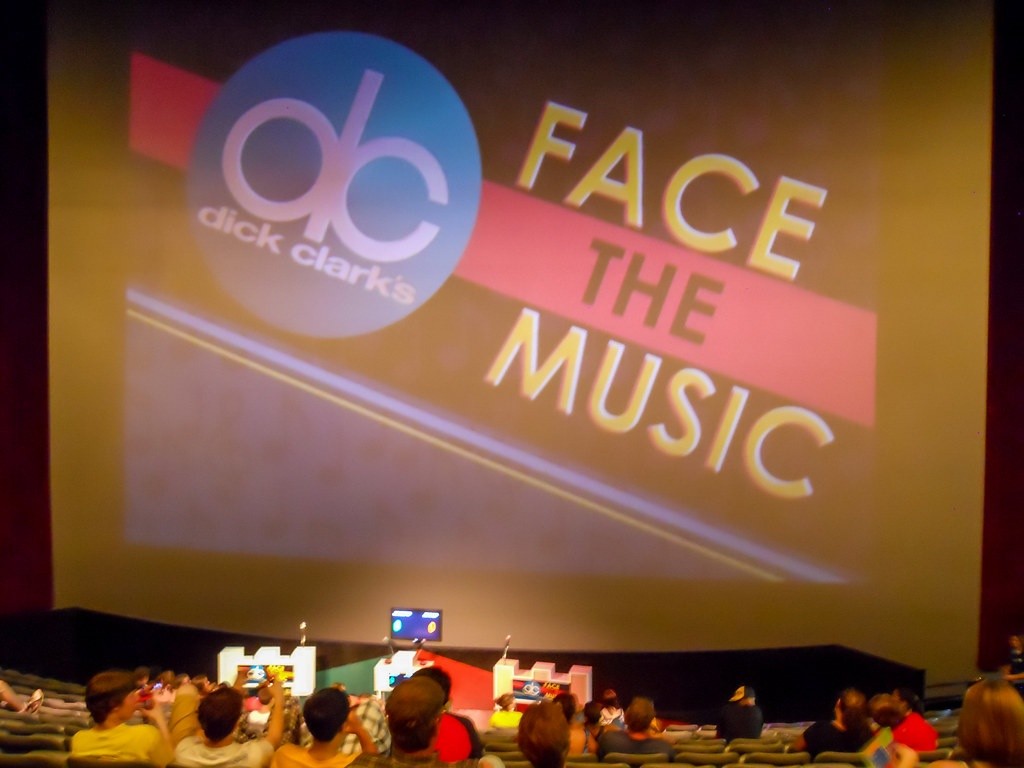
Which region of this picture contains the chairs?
[0,669,966,768]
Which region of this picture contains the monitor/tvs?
[390,608,441,642]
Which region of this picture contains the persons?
[477,701,571,768]
[870,693,939,768]
[168,664,302,768]
[598,688,624,732]
[552,694,586,757]
[271,682,393,768]
[71,666,175,768]
[0,680,44,714]
[886,675,1024,768]
[598,694,675,765]
[999,630,1024,699]
[792,689,874,768]
[412,666,485,760]
[582,702,620,754]
[713,687,764,745]
[489,693,523,731]
[344,676,478,768]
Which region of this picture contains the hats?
[386,678,445,729]
[729,686,756,701]
